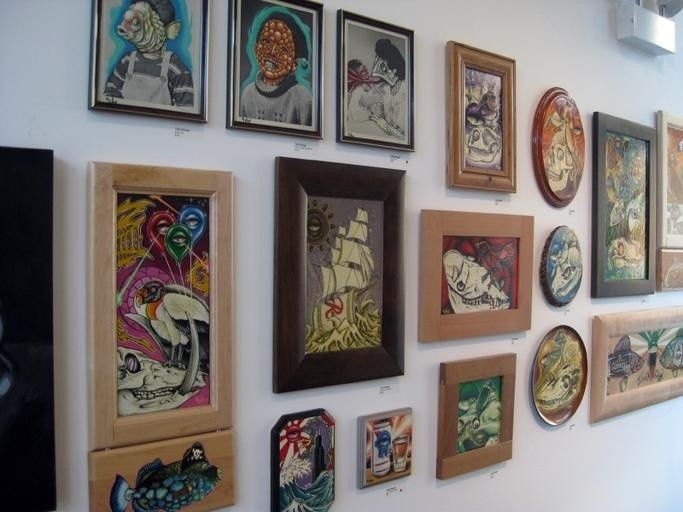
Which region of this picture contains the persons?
[239,7,312,127]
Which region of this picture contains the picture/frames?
[591,304,682,424]
[416,207,535,346]
[228,2,324,140]
[83,159,233,452]
[448,37,520,202]
[334,12,418,155]
[86,2,208,124]
[435,353,517,482]
[589,110,656,299]
[0,146,58,512]
[651,106,682,252]
[273,158,407,402]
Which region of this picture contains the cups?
[391,436,411,472]
